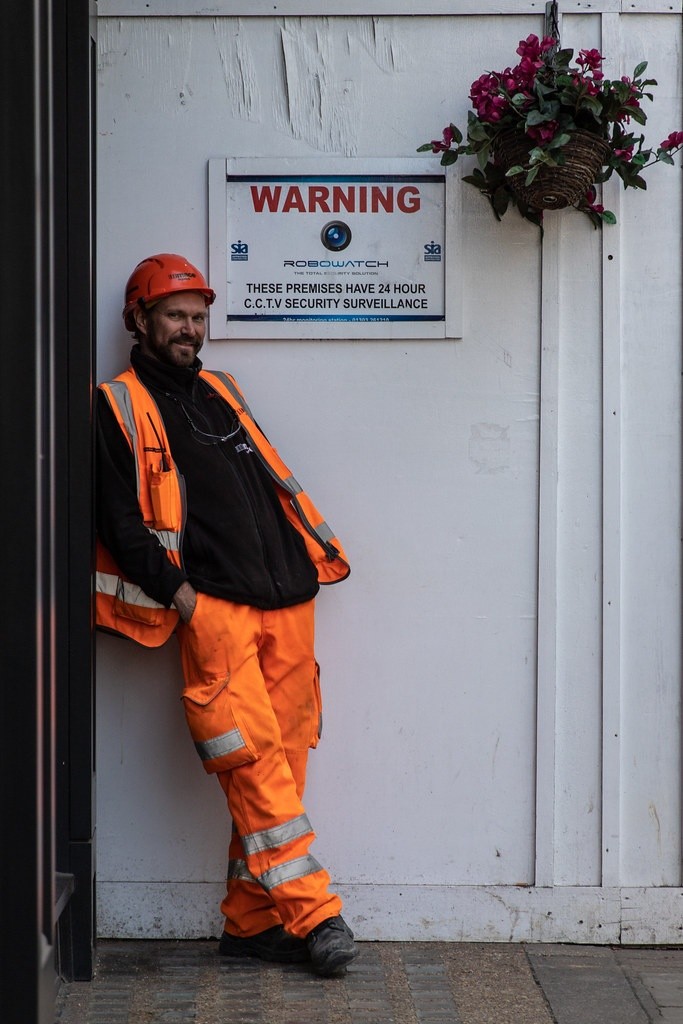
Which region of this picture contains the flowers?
[417,33,683,242]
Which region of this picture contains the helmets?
[122,253,215,318]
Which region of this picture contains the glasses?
[174,391,242,446]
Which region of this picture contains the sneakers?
[306,912,359,980]
[217,923,306,964]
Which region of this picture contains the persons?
[94,253,360,976]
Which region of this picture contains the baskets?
[491,124,610,211]
